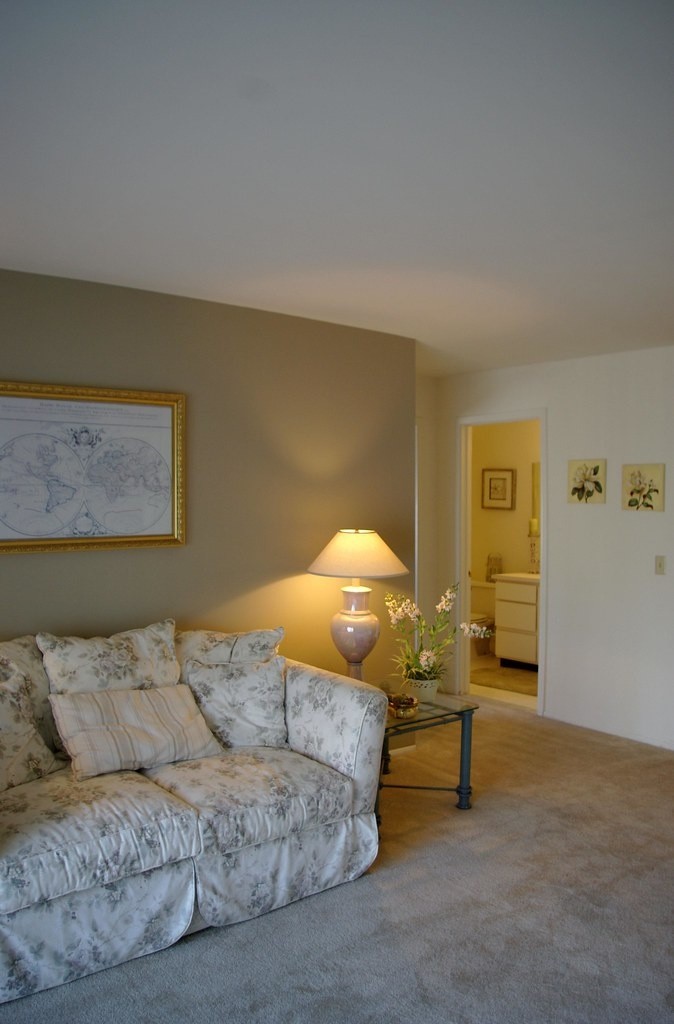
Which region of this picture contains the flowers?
[385,582,497,693]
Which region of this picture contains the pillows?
[174,629,288,673]
[36,618,183,696]
[188,656,292,752]
[50,685,225,784]
[0,634,66,794]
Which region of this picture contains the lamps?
[306,528,410,683]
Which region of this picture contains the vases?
[402,677,440,703]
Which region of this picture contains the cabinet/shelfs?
[491,572,540,666]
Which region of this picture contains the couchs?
[0,658,390,1005]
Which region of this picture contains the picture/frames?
[0,381,189,556]
[481,468,516,511]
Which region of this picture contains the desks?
[374,693,480,843]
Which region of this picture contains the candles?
[529,518,539,534]
